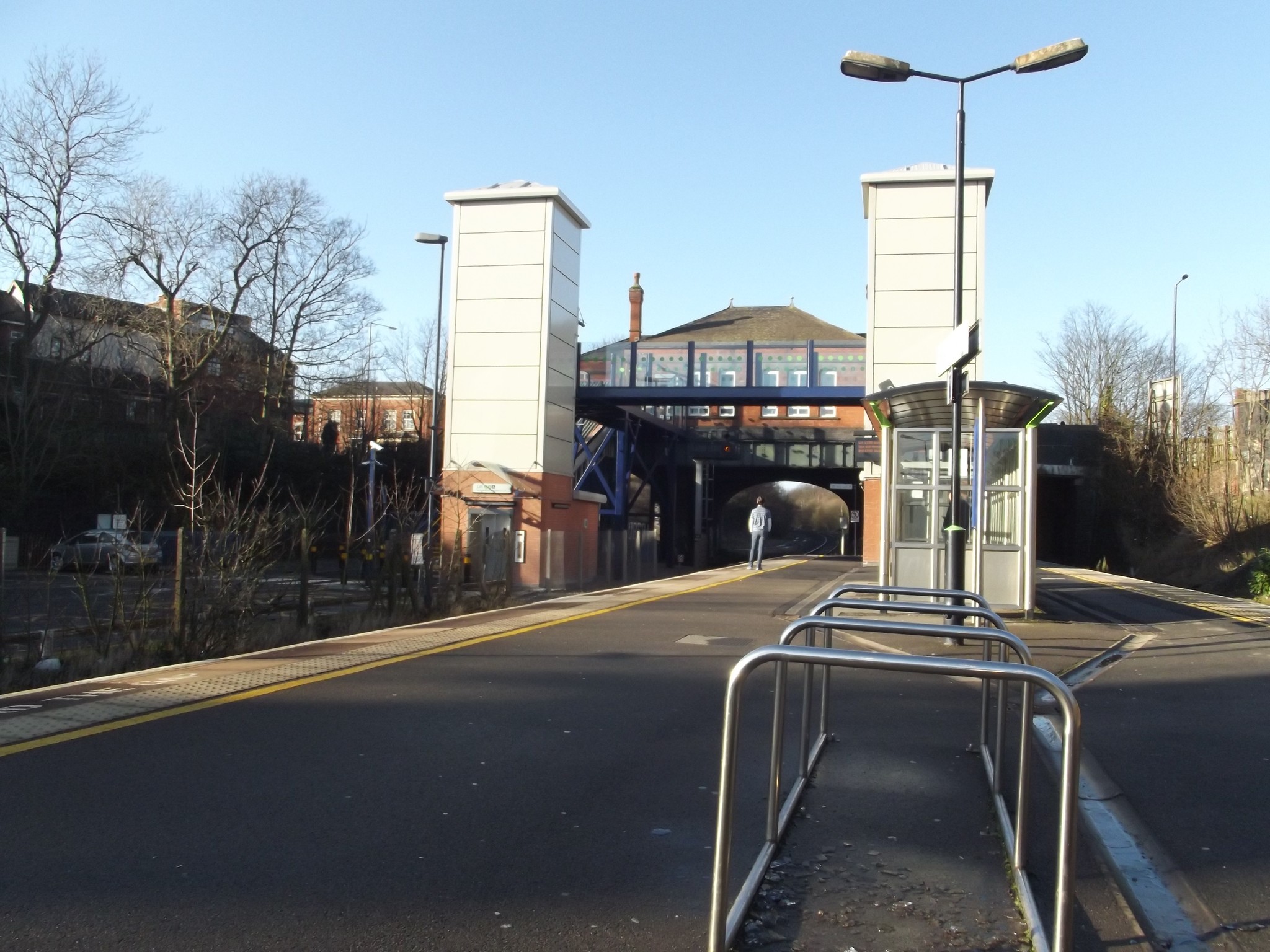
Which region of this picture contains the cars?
[47,528,163,578]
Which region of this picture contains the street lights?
[1170,273,1189,473]
[364,322,398,431]
[413,230,448,549]
[839,36,1088,645]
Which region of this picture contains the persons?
[747,496,772,570]
